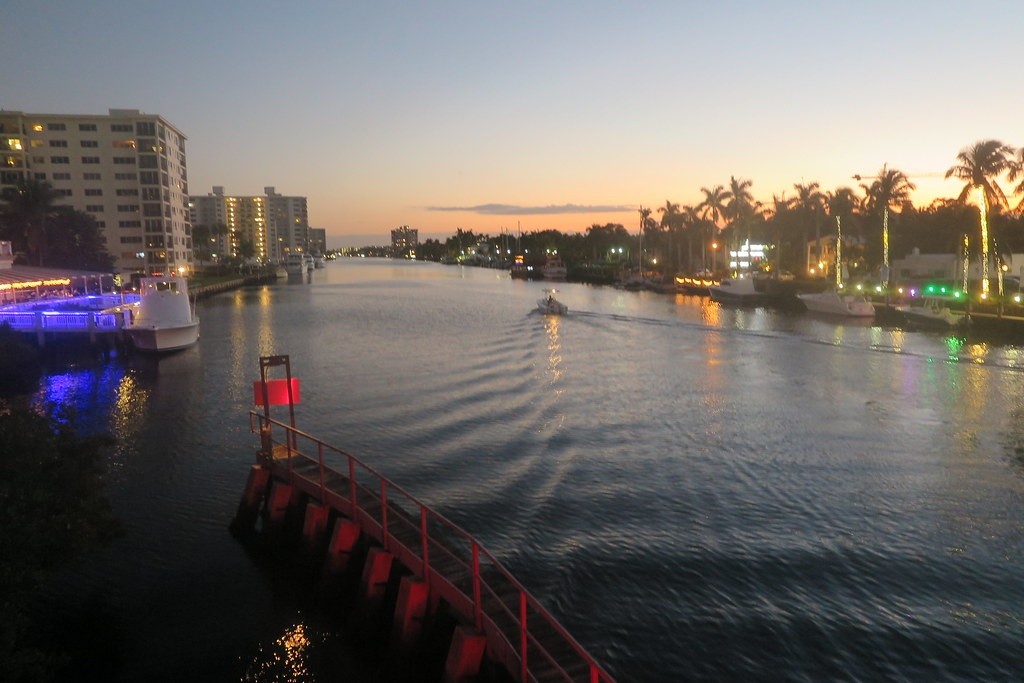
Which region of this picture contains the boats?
[794,286,877,317]
[510,254,567,279]
[707,277,770,305]
[286,253,326,274]
[121,276,201,353]
[536,288,569,316]
[894,296,974,331]
